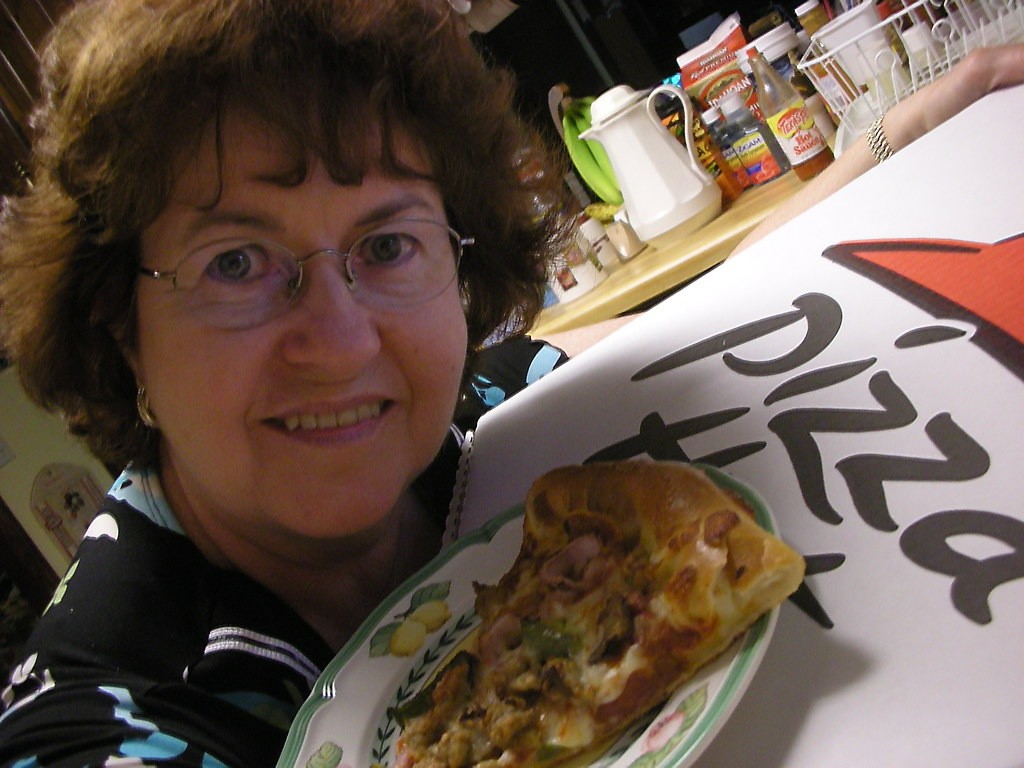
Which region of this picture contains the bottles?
[902,22,947,75]
[794,1,868,101]
[746,46,836,181]
[877,2,923,68]
[788,50,841,127]
[508,148,611,305]
[701,107,755,175]
[791,70,838,139]
[719,91,791,188]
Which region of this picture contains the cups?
[735,22,836,140]
[810,1,911,109]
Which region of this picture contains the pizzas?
[394,458,807,768]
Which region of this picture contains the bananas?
[560,96,626,208]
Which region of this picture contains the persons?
[0,0,1024,768]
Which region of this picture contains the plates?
[275,463,780,768]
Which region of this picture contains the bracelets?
[866,114,893,165]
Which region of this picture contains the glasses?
[134,215,478,332]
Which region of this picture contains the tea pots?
[577,84,723,250]
